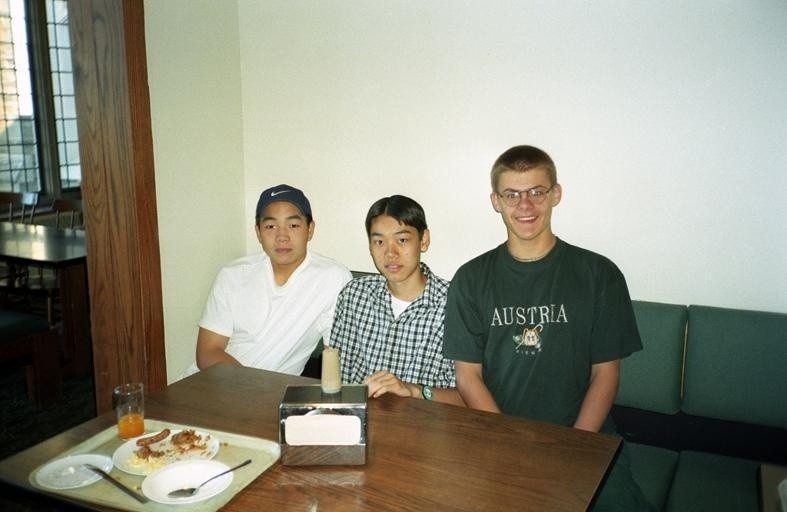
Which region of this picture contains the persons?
[328,197,471,407]
[441,145,642,433]
[196,183,357,374]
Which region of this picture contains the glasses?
[495,183,556,204]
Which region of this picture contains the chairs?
[0,192,89,402]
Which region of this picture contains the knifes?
[85,461,148,505]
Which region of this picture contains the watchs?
[415,382,435,402]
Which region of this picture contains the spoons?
[163,460,253,497]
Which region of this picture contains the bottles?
[319,347,342,395]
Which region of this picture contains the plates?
[113,428,221,474]
[140,462,235,504]
[36,455,114,491]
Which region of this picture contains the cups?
[113,383,145,439]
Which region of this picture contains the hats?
[257,185,311,220]
